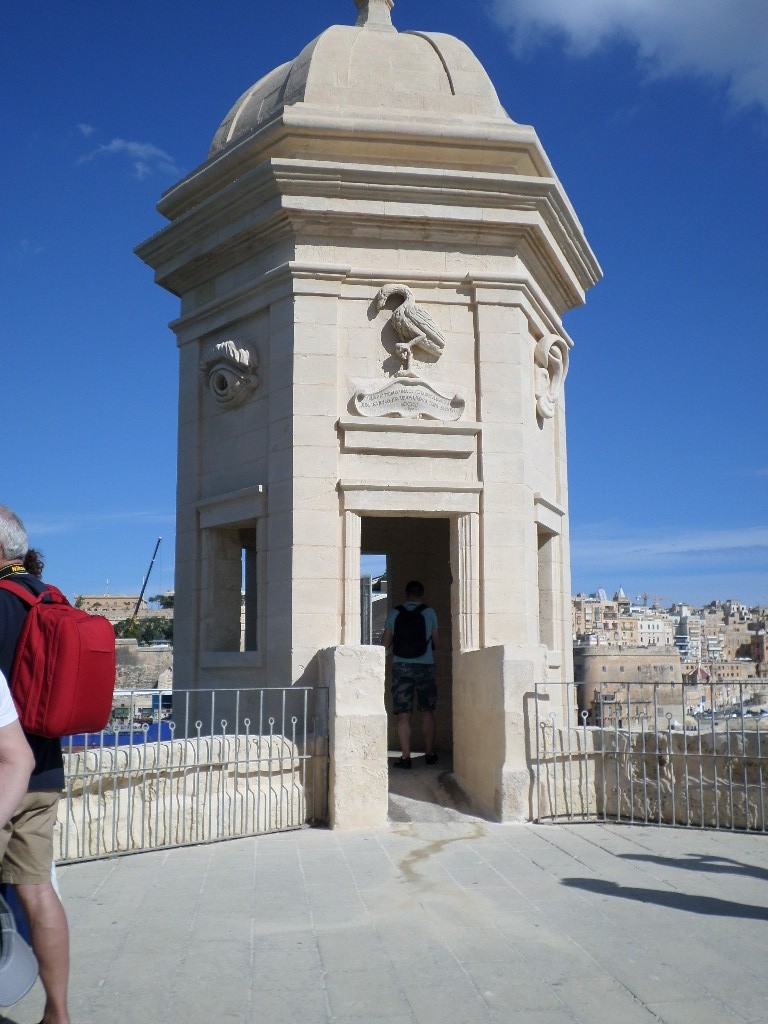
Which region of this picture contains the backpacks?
[1,578,115,739]
[391,604,432,658]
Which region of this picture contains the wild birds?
[377,283,446,359]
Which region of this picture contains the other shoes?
[425,753,438,765]
[394,757,411,769]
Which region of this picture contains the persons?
[0,503,70,1024]
[382,580,443,768]
